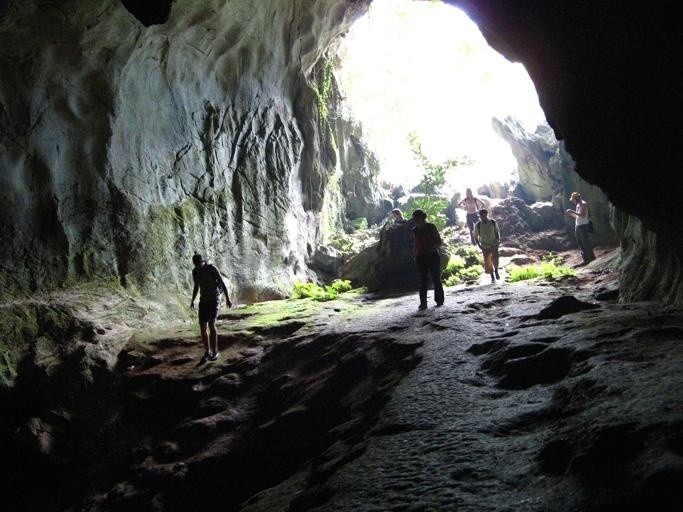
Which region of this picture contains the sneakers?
[199,350,220,364]
[418,301,444,308]
[491,274,500,284]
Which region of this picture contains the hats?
[568,191,581,202]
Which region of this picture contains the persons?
[390,209,408,224]
[473,209,501,282]
[411,208,450,309]
[188,254,232,363]
[564,192,596,269]
[457,188,485,246]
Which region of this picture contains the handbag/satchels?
[586,221,594,233]
